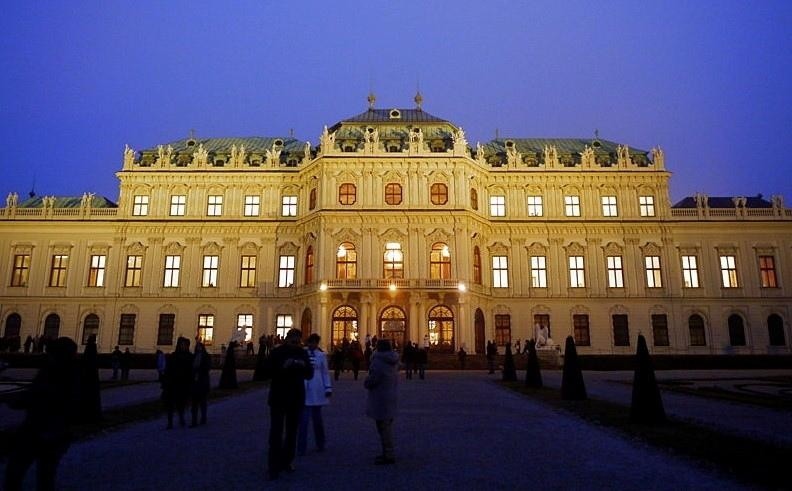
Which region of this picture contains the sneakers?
[166,417,209,430]
[333,369,425,381]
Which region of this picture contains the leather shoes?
[266,443,399,480]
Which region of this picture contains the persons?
[360,340,400,468]
[333,337,373,382]
[486,339,542,391]
[188,343,214,430]
[457,346,468,369]
[1,338,100,491]
[8,333,44,356]
[300,333,334,458]
[247,334,286,359]
[401,341,428,381]
[159,336,197,433]
[109,344,132,383]
[156,350,166,382]
[263,328,314,483]
